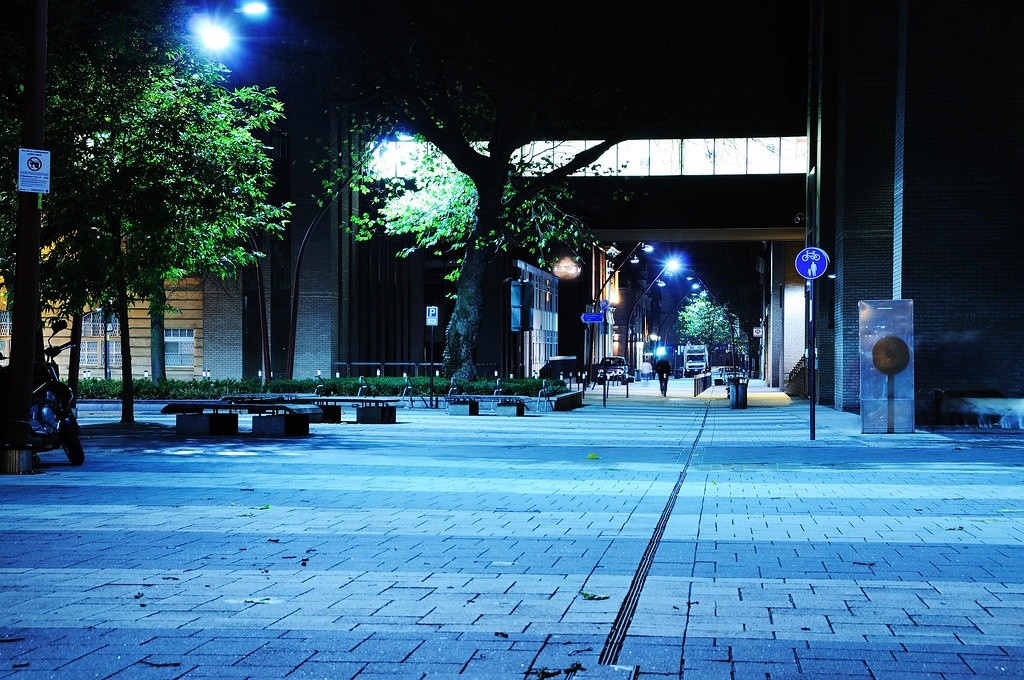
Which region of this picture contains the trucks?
[684,345,711,375]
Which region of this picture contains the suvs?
[597,357,628,381]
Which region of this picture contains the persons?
[655,354,671,397]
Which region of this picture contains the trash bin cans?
[727,376,749,410]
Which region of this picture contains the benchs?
[161,393,401,438]
[443,394,533,417]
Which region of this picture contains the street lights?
[655,283,699,359]
[625,259,678,355]
[587,241,654,384]
[289,126,413,382]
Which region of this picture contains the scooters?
[0,321,85,466]
